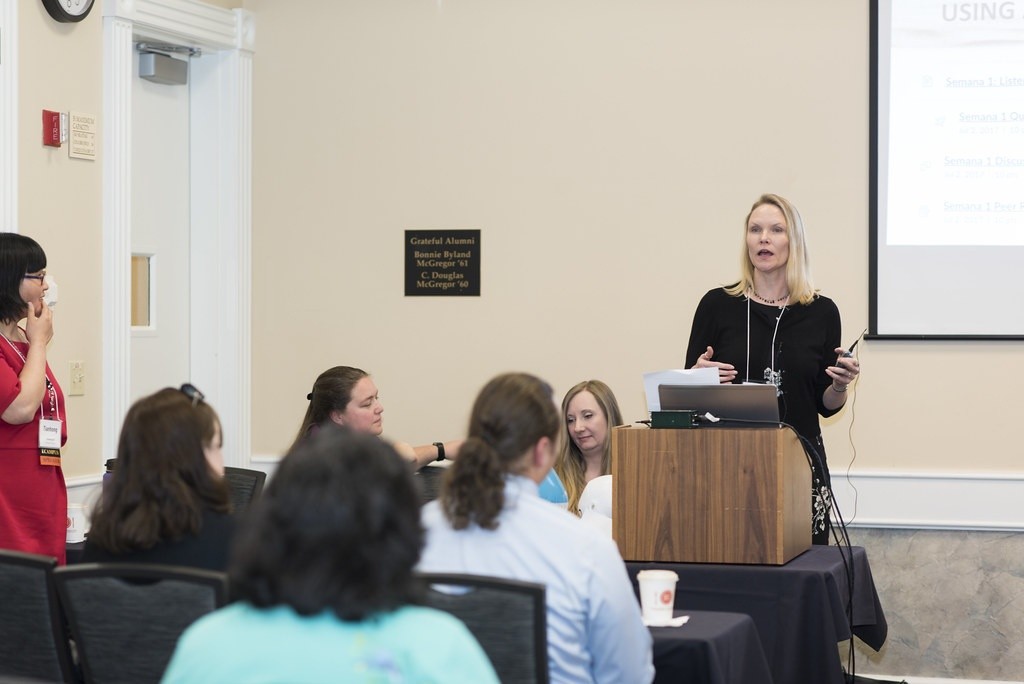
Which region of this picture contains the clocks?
[41,0,95,23]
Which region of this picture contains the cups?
[637,570,679,619]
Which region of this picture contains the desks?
[622,545,888,684]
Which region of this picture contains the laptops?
[658,383,780,430]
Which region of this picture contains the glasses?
[181,384,205,407]
[22,269,47,285]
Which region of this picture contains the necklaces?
[0,328,56,413]
[747,283,790,303]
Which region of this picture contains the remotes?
[837,353,852,369]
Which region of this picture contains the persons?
[553,379,623,519]
[685,193,857,545]
[410,373,657,684]
[79,387,235,573]
[158,425,503,684]
[291,366,462,475]
[0,232,84,684]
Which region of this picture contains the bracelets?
[432,442,445,461]
[831,383,848,392]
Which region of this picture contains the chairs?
[0,459,543,683]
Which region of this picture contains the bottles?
[102,457,119,484]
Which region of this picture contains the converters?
[650,411,699,430]
